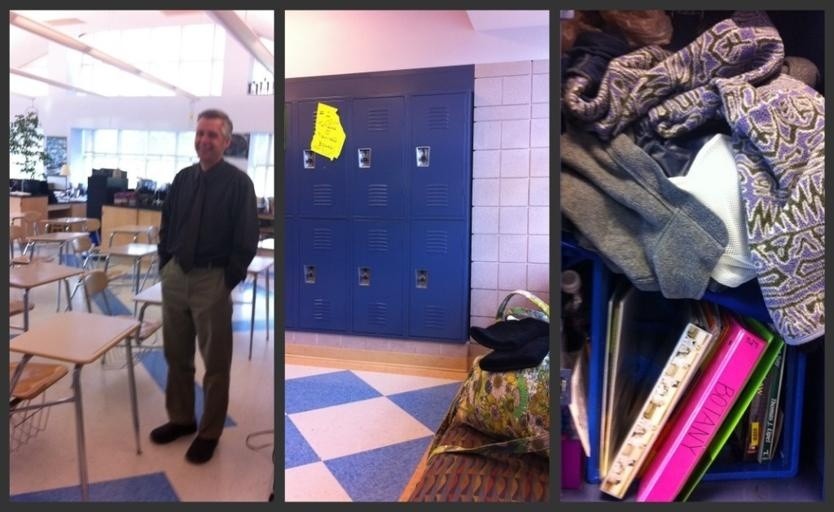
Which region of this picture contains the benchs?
[394,347,549,502]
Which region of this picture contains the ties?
[175,174,206,273]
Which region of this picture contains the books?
[589,287,787,503]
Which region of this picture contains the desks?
[8,186,275,502]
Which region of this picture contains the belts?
[196,259,228,268]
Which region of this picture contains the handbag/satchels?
[451,290,551,462]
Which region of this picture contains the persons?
[150,108,258,464]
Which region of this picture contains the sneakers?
[151,421,197,442]
[185,436,218,463]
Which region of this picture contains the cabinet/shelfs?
[285,91,471,342]
